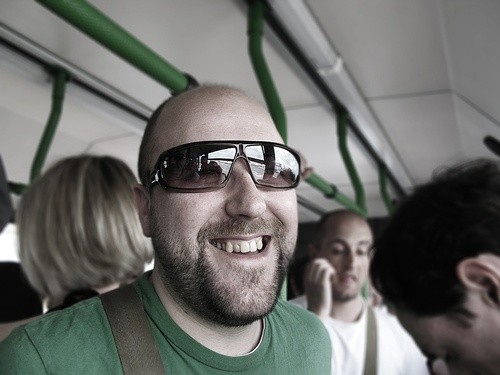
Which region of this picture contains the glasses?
[144,139,300,194]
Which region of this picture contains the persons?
[366,158,500,375]
[0,85,331,375]
[0,155,155,341]
[287,208,430,375]
[171,156,222,183]
